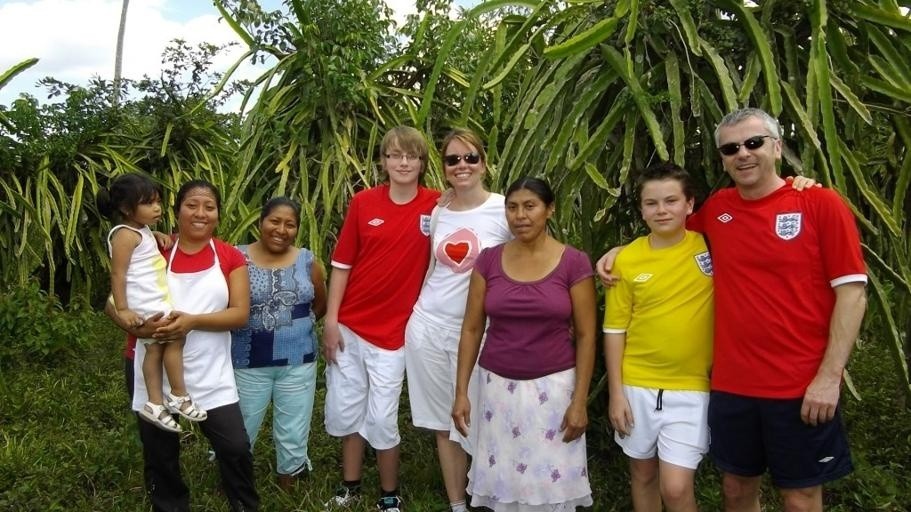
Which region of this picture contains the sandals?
[140,392,211,433]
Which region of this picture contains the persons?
[104,181,261,512]
[449,177,596,512]
[404,129,509,512]
[601,163,821,511]
[322,125,442,512]
[96,173,208,435]
[595,109,868,512]
[228,196,329,492]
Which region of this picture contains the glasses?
[383,150,424,162]
[718,135,775,156]
[443,152,482,166]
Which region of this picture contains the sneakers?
[324,487,364,510]
[377,495,404,512]
